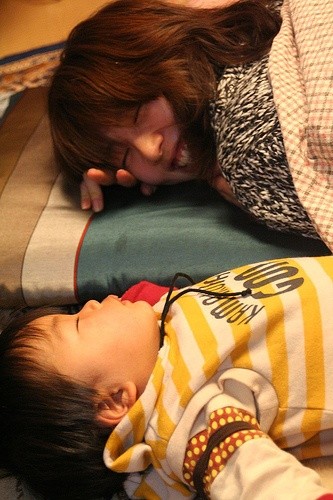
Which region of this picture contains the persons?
[0,256,333,499]
[47,0,321,241]
